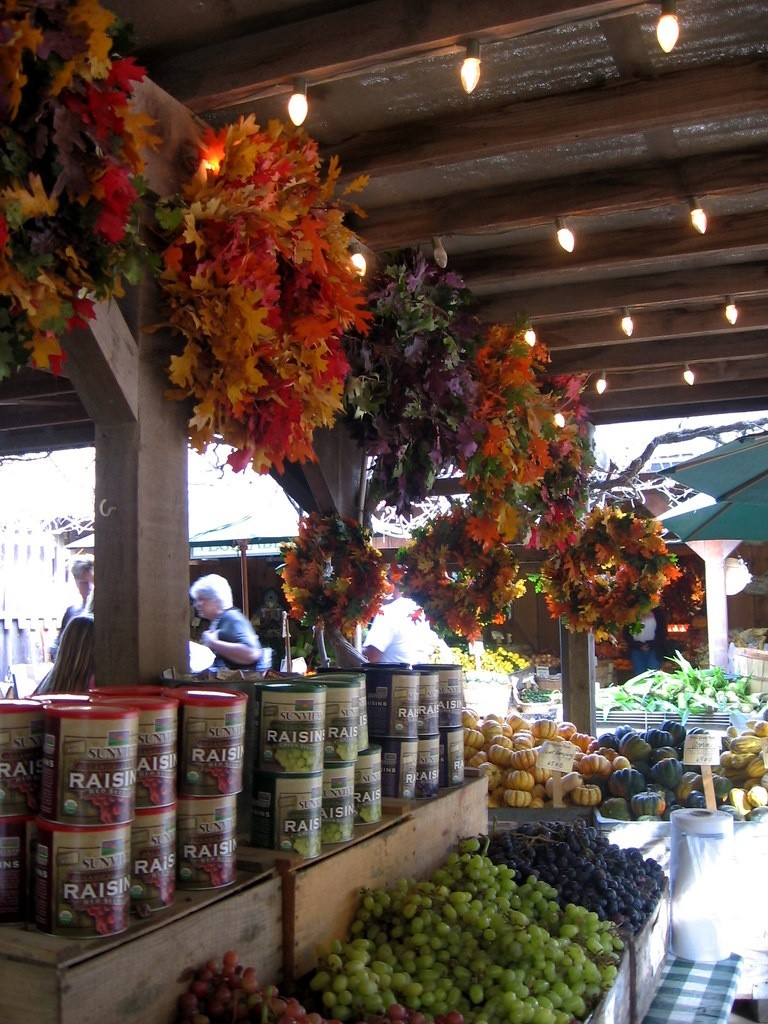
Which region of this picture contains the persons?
[621,609,667,678]
[32,553,98,694]
[363,565,431,663]
[190,572,266,677]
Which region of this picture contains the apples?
[520,653,559,666]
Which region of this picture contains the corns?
[595,670,768,714]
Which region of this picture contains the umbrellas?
[629,432,768,542]
[65,490,411,620]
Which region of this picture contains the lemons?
[450,646,530,674]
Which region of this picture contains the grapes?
[178,815,667,1024]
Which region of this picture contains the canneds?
[0,661,464,939]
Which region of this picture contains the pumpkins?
[461,706,768,822]
